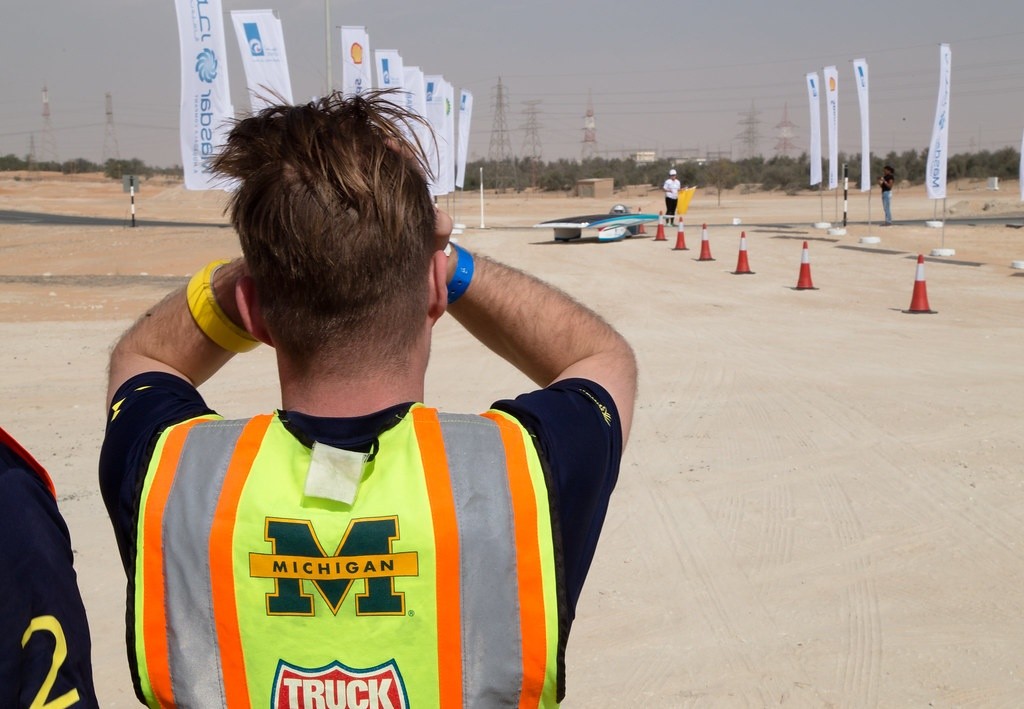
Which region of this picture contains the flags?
[174,0,473,195]
[805,44,951,200]
[678,188,695,215]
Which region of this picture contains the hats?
[669,169,677,175]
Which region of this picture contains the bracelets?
[187,258,263,352]
[444,242,473,303]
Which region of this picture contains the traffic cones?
[795,241,819,290]
[673,217,687,251]
[734,232,753,273]
[654,211,667,242]
[697,225,714,259]
[637,208,645,235]
[906,253,937,313]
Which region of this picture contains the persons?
[0,426,99,709]
[663,169,683,227]
[879,166,895,224]
[97,96,637,709]
[615,206,625,213]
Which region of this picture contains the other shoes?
[671,224,674,226]
[666,223,670,226]
